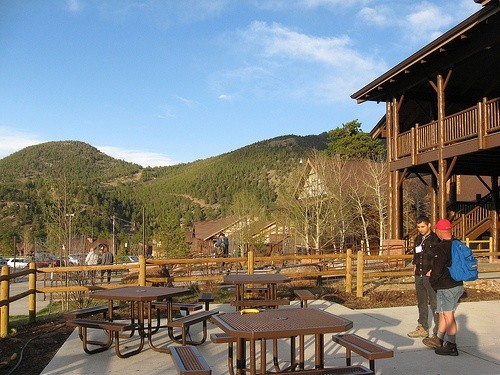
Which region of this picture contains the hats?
[431,219,452,230]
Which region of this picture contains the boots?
[406,323,429,337]
[422,333,443,348]
[431,332,437,338]
[435,341,458,355]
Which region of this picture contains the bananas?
[240,308,259,315]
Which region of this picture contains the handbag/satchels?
[216,243,225,255]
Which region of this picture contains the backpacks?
[444,238,478,282]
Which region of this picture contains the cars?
[7,257,29,269]
[0,257,7,268]
[31,256,81,267]
[123,256,140,262]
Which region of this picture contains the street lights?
[65,213,76,266]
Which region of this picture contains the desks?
[223,274,291,311]
[89,286,193,338]
[210,308,354,375]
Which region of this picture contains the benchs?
[64,289,395,374]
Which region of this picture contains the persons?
[407,215,439,338]
[85,248,98,277]
[217,233,229,273]
[423,219,465,356]
[101,248,114,284]
